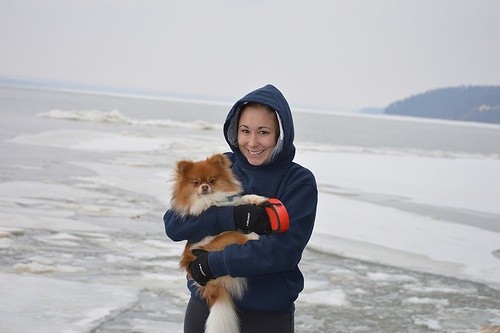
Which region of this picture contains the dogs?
[166,153,268,333]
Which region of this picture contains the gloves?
[185,249,216,287]
[233,200,274,240]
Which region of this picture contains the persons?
[163,85,318,333]
